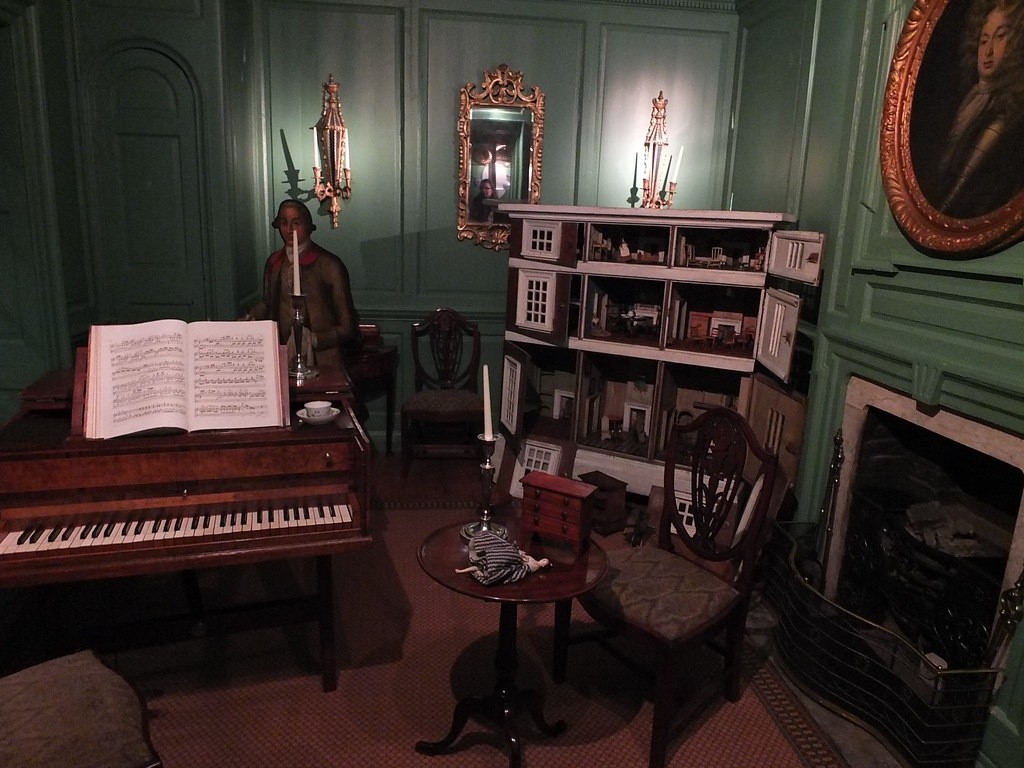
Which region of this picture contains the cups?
[304,401,332,417]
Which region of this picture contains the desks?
[415,523,610,756]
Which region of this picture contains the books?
[85,320,284,441]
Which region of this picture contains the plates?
[296,407,341,426]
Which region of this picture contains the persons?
[471,179,511,224]
[236,200,360,366]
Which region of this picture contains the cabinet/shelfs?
[495,204,829,546]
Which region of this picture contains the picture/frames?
[878,0,1024,257]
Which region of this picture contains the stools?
[0,647,161,768]
[346,345,397,459]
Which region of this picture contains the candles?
[672,146,684,182]
[645,140,653,179]
[483,365,493,440]
[293,229,300,295]
[345,128,351,169]
[313,127,318,166]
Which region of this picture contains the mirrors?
[455,63,545,251]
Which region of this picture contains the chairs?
[400,307,485,476]
[554,406,780,768]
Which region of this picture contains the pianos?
[0,347,379,691]
